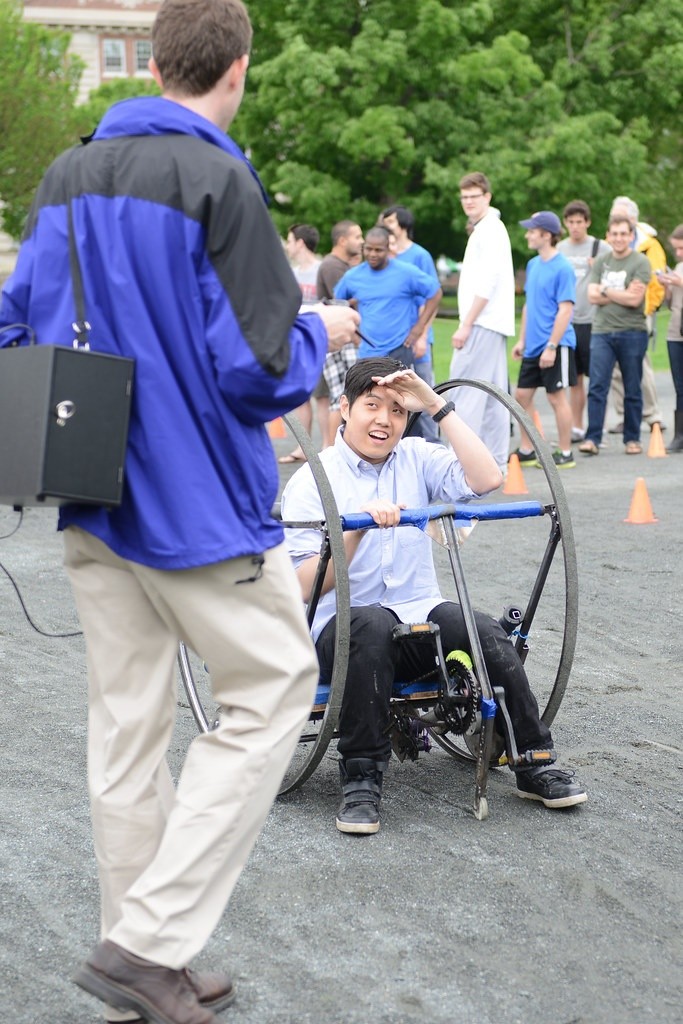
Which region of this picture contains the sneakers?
[336,758,380,833]
[507,447,537,467]
[516,767,588,807]
[538,449,576,468]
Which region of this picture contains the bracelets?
[432,400,455,423]
[602,286,608,297]
[548,343,556,350]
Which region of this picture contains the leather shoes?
[72,939,222,1024]
[185,966,236,1012]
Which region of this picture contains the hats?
[519,211,561,234]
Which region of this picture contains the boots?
[665,411,683,453]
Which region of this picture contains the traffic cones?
[624,478,658,523]
[647,423,669,456]
[503,455,529,494]
[534,412,545,441]
[269,416,285,439]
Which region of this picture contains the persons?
[281,357,588,831]
[507,209,577,469]
[555,199,614,448]
[278,223,330,463]
[316,220,364,445]
[383,205,440,444]
[335,227,443,438]
[609,195,667,434]
[448,171,515,476]
[578,217,652,454]
[657,222,683,453]
[0,0,361,1024]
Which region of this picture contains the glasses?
[459,195,483,200]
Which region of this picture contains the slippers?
[277,454,305,464]
[625,441,641,454]
[579,440,599,455]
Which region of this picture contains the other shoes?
[608,423,624,434]
[571,427,586,443]
[649,420,666,433]
